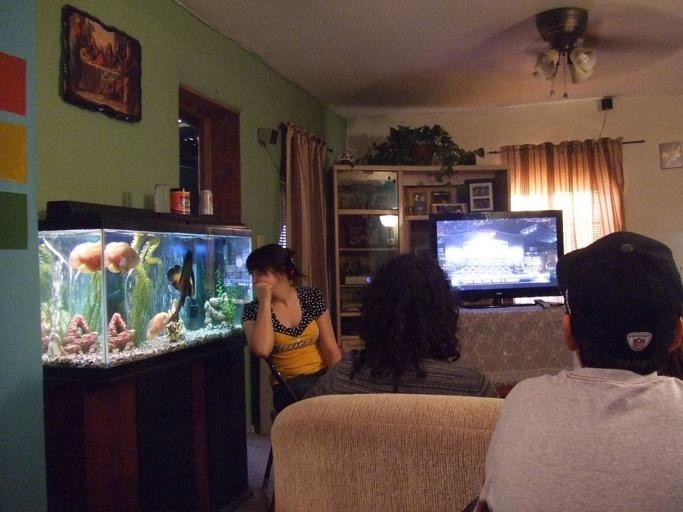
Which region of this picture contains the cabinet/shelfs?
[327,165,511,352]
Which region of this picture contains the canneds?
[198,189,214,215]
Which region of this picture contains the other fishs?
[102,242,140,274]
[146,299,181,340]
[167,250,196,324]
[69,241,102,274]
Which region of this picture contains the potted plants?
[363,119,485,183]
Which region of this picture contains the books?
[340,275,371,352]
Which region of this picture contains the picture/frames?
[404,178,495,215]
[659,141,683,169]
[57,4,142,124]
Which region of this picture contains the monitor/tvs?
[426,210,566,307]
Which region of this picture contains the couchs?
[270,393,505,511]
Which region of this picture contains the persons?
[472,229,682,511]
[301,253,502,400]
[241,243,342,412]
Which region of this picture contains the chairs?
[261,358,299,512]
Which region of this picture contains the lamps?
[532,8,598,100]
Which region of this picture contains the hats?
[557,231,682,360]
